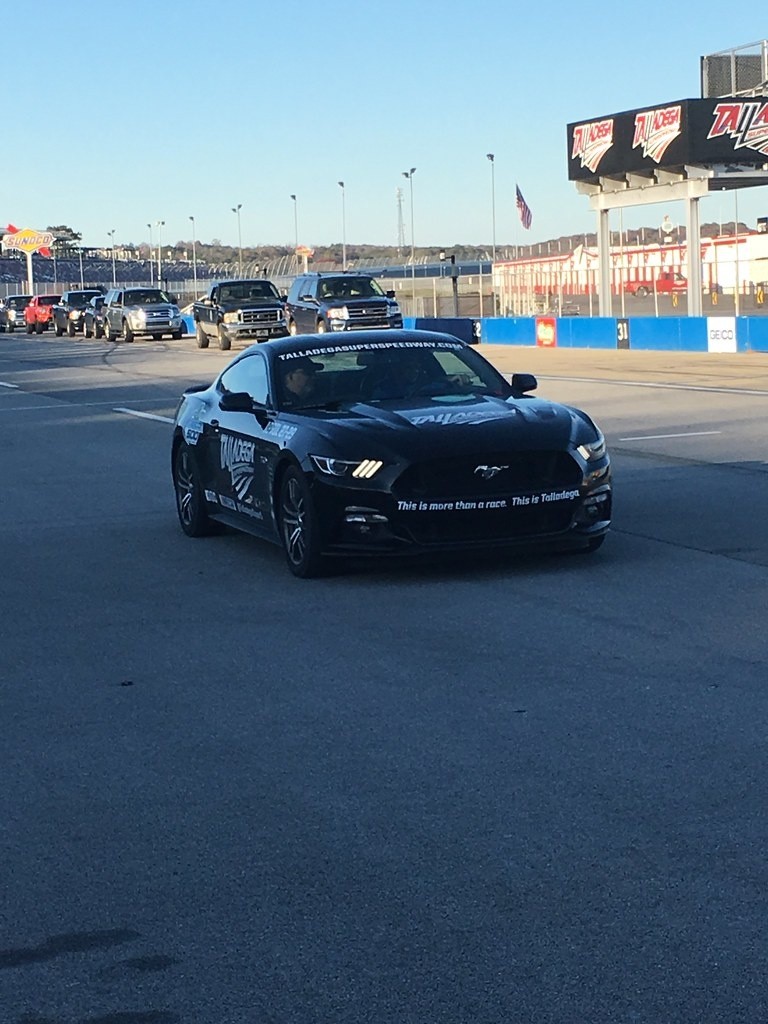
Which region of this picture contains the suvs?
[99,286,183,342]
[283,271,404,356]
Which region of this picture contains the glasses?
[294,370,314,376]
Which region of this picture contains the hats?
[278,357,324,376]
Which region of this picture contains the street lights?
[190,216,198,300]
[291,195,298,277]
[74,242,83,291]
[158,275,167,292]
[107,230,116,287]
[338,181,345,271]
[51,246,56,295]
[254,264,266,280]
[147,224,153,287]
[402,168,416,316]
[440,248,458,316]
[156,221,165,289]
[487,155,496,318]
[232,204,243,279]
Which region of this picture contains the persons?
[374,352,470,398]
[281,358,324,406]
[221,287,234,299]
[145,293,158,303]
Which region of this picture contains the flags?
[516,186,531,230]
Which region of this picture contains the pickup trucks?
[193,279,287,351]
[23,294,63,334]
[625,272,707,299]
[0,295,33,333]
[51,289,102,337]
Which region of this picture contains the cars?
[83,296,106,339]
[178,294,208,335]
[0,298,17,308]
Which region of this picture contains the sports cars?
[171,329,613,580]
